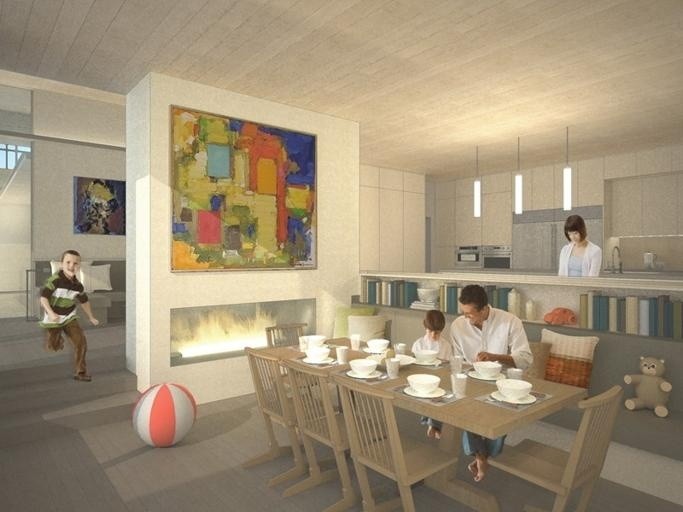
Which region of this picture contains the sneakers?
[71,368,93,381]
